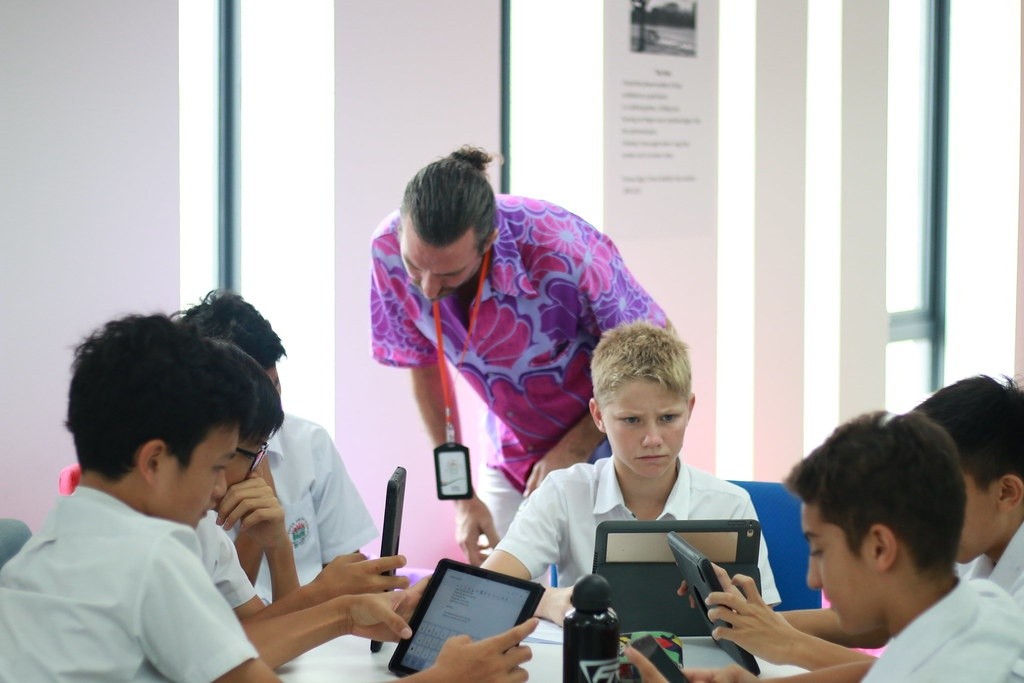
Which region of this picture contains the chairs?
[552,480,821,612]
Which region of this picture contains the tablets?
[666,530,761,677]
[593,520,761,637]
[370,467,406,653]
[387,559,546,676]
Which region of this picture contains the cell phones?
[630,634,690,683]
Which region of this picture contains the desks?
[271,617,816,683]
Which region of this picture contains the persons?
[195,338,409,622]
[169,289,379,607]
[1,313,539,683]
[370,145,677,568]
[475,321,784,627]
[678,373,1024,668]
[626,411,1024,683]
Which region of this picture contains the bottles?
[563,575,620,683]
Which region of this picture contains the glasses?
[235,442,269,480]
